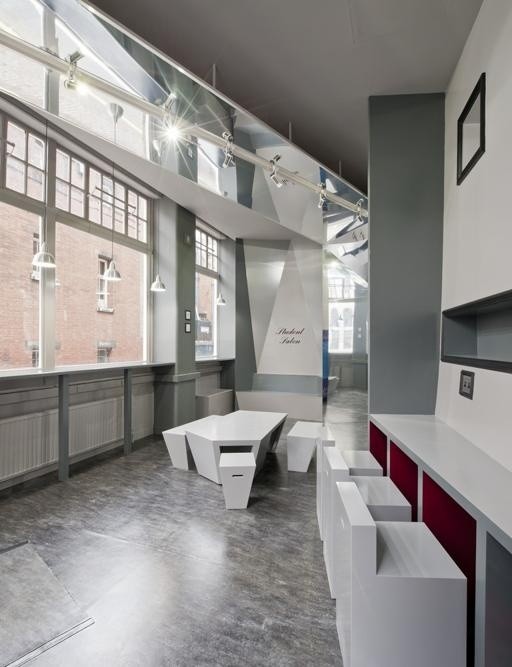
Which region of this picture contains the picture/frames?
[458,369,474,400]
[185,310,191,333]
[457,73,487,186]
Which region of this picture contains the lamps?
[31,66,166,294]
[215,236,226,305]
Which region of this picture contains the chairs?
[316,427,468,666]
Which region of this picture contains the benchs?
[162,410,322,510]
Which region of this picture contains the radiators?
[196,374,222,403]
[0,395,134,484]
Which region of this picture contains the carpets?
[1,539,97,667]
